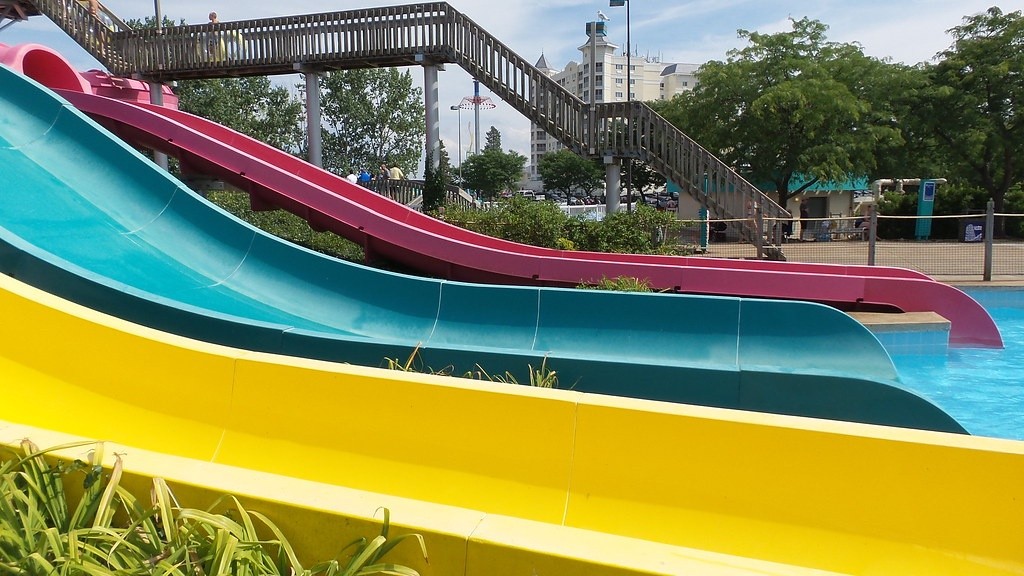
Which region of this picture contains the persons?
[585,193,606,205]
[347,163,404,202]
[847,204,880,242]
[207,11,220,62]
[773,199,809,243]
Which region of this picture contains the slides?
[0,59,1024,576]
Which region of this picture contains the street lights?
[609,0,632,214]
[450,106,462,203]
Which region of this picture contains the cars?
[499,187,679,204]
